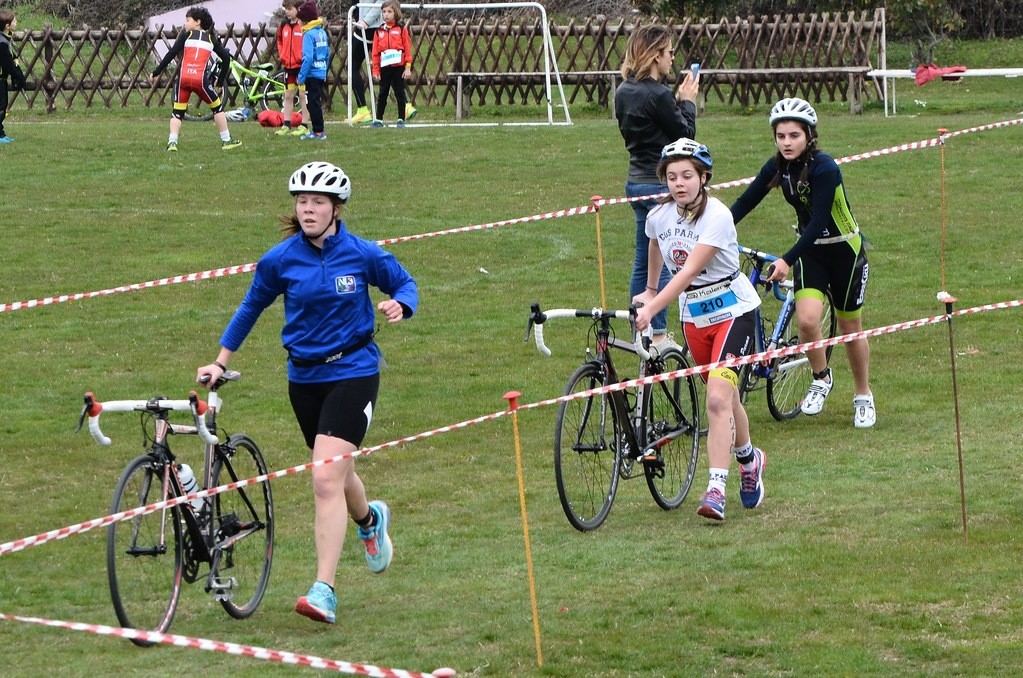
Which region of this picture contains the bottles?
[176,463,204,512]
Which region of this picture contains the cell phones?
[691,64,700,82]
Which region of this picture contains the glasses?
[660,48,674,57]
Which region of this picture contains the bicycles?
[169,48,309,122]
[72,370,275,649]
[672,245,838,439]
[523,302,700,533]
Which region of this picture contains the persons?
[150,4,242,151]
[729,98,876,427]
[197,162,417,621]
[0,10,26,142]
[615,26,700,357]
[276,0,330,139]
[344,0,418,126]
[631,138,768,521]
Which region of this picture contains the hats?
[295,0,317,21]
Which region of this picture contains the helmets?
[770,98,818,125]
[225,109,245,122]
[288,162,352,205]
[662,137,713,181]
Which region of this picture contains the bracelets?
[214,361,226,372]
[646,286,659,292]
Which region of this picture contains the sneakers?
[295,582,337,624]
[345,106,372,123]
[274,125,290,136]
[369,120,383,128]
[853,391,876,428]
[396,119,405,128]
[0,135,14,143]
[739,447,766,509]
[801,368,833,414]
[649,334,691,358]
[405,102,417,119]
[222,138,242,150]
[358,500,392,573]
[165,142,177,151]
[300,131,327,139]
[291,124,311,136]
[696,488,725,520]
